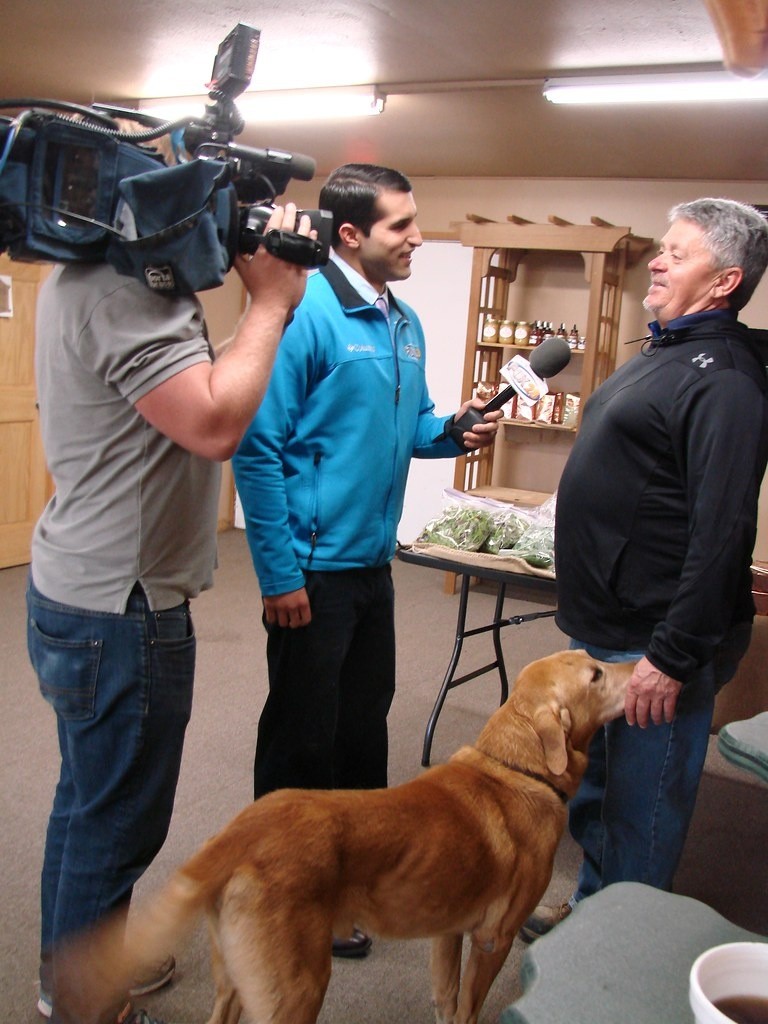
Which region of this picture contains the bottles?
[528,321,585,349]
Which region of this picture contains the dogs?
[46,647,640,1023]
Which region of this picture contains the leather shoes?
[331,928,372,957]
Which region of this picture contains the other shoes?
[520,903,572,940]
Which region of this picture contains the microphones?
[224,141,316,181]
[477,337,571,420]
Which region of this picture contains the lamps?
[541,69,768,100]
[137,84,388,122]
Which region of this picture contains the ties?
[375,301,392,328]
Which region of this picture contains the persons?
[25,104,316,1024]
[232,163,504,956]
[516,197,768,943]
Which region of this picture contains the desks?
[398,540,560,768]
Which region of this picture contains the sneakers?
[121,950,176,996]
[38,992,165,1024]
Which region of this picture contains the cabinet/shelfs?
[444,213,655,598]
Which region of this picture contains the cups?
[689,941,767,1023]
